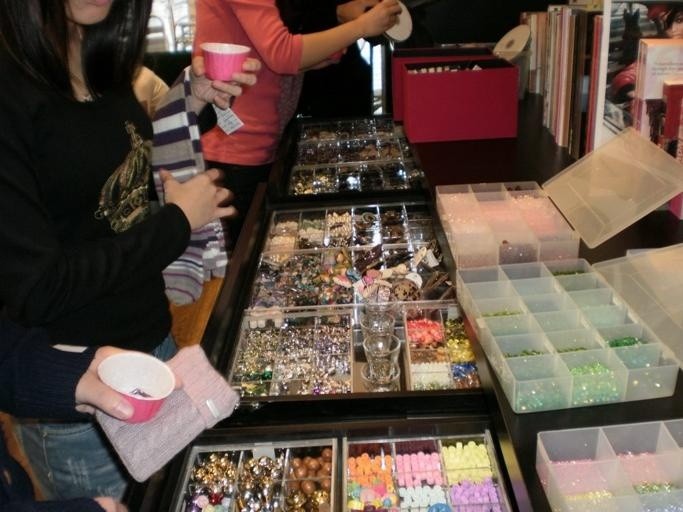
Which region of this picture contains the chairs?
[147,16,164,42]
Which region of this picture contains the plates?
[383,2,413,43]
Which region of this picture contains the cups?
[360,295,402,391]
[199,42,251,85]
[98,352,175,424]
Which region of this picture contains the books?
[631,36,683,219]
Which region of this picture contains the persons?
[194,1,402,259]
[615,4,683,100]
[0,1,235,512]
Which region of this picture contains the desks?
[143,37,192,90]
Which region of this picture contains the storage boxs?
[402,58,519,143]
[390,49,493,122]
